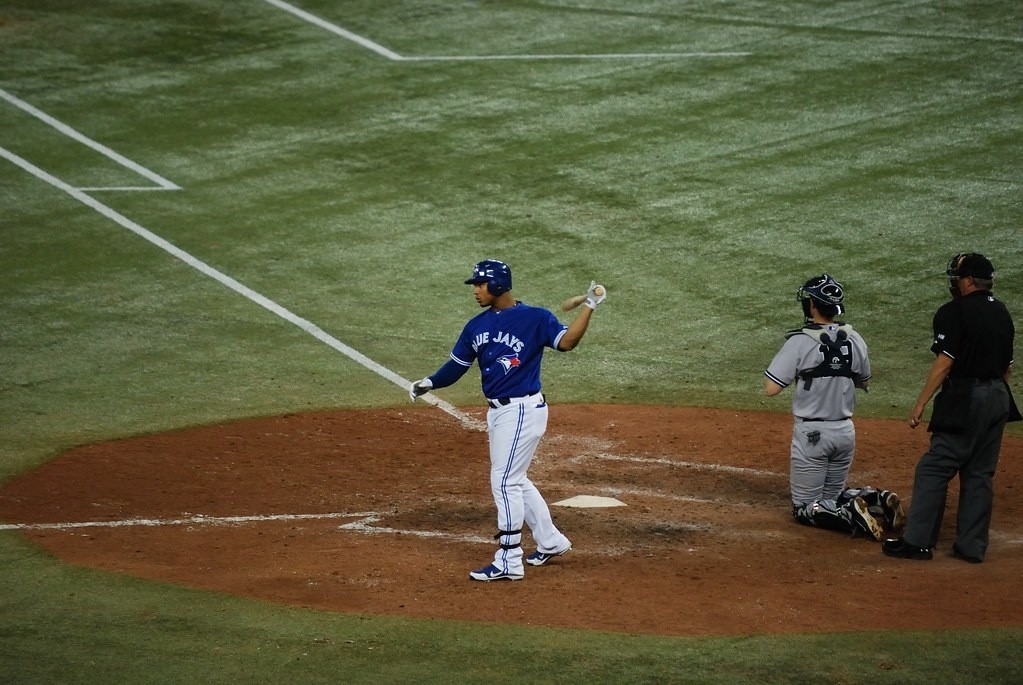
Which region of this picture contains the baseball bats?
[561,288,604,311]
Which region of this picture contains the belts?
[803,418,849,422]
[971,376,1001,383]
[487,391,539,409]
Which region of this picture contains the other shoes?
[882,540,934,559]
[953,543,983,563]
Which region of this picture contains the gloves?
[410,377,433,403]
[585,281,607,310]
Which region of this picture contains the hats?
[947,253,994,280]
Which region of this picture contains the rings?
[911,419,914,423]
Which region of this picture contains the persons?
[880,250,1016,565]
[408,258,609,582]
[763,272,909,545]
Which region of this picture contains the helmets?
[464,259,512,296]
[797,274,844,325]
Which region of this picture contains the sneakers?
[843,497,884,541]
[470,564,525,581]
[879,489,906,531]
[526,542,572,566]
